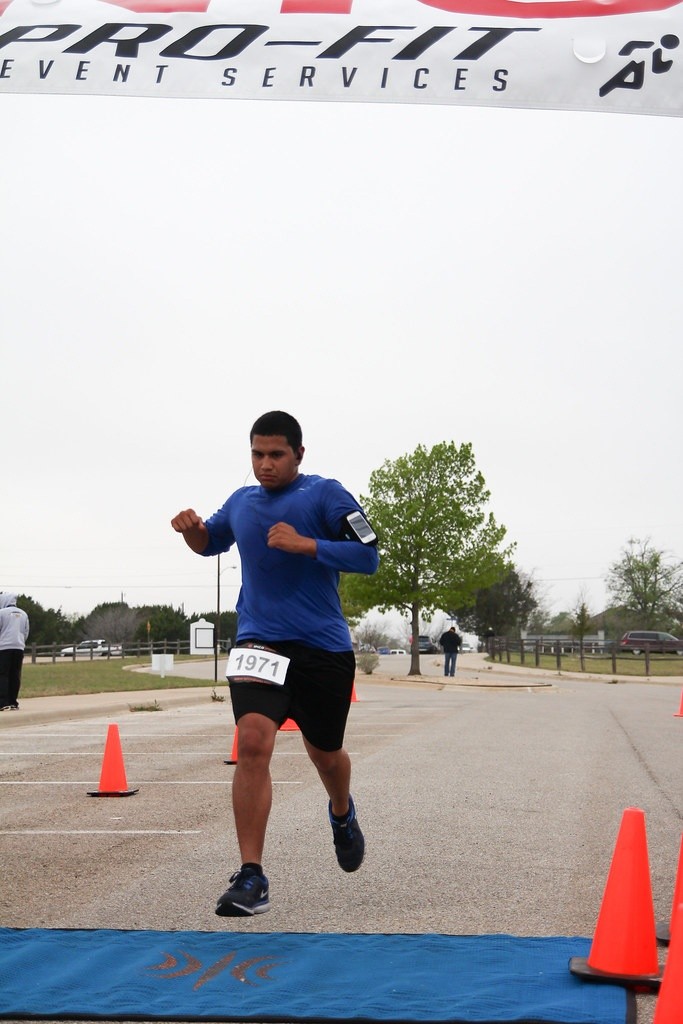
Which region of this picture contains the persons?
[0,592,31,713]
[439,627,462,676]
[170,410,380,919]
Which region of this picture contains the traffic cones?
[279,718,300,731]
[650,904,683,1024]
[351,681,361,702]
[569,805,667,988]
[86,722,139,797]
[673,688,683,717]
[656,833,683,942]
[224,725,239,764]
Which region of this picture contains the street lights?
[216,565,238,654]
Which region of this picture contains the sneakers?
[216,872,270,916]
[329,794,365,871]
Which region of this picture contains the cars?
[359,645,408,655]
[458,643,474,653]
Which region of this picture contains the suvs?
[412,635,438,654]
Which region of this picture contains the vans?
[619,630,683,656]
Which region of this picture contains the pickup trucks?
[61,639,125,657]
